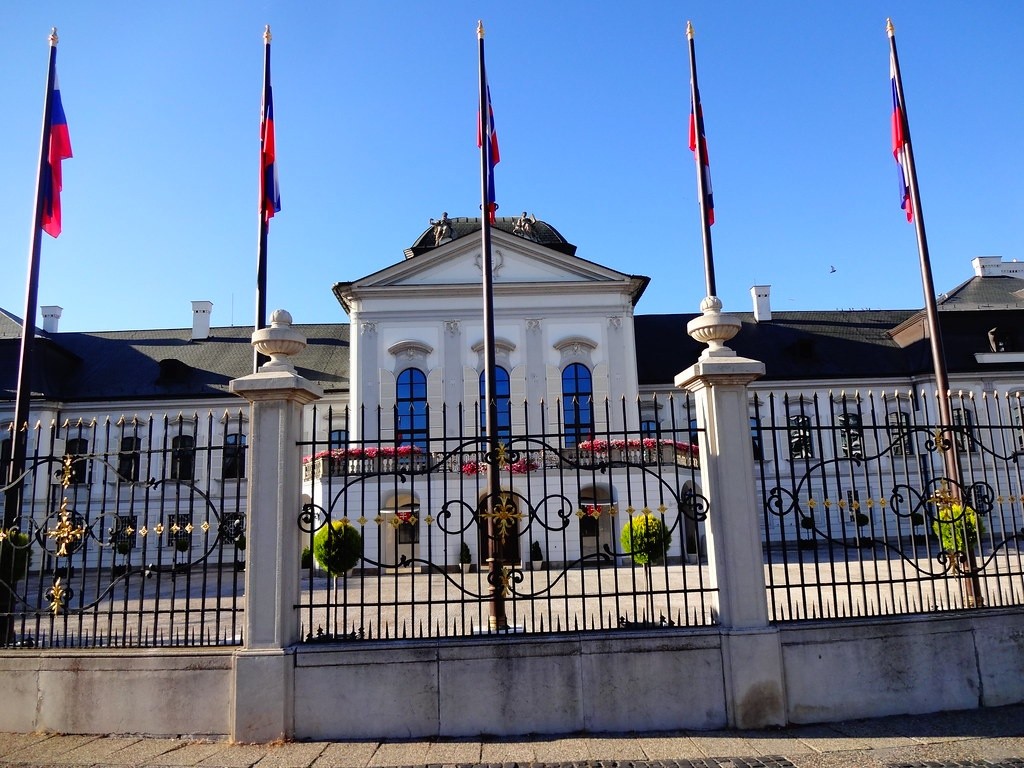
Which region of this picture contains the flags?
[258,83,280,230]
[478,72,500,224]
[688,76,715,226]
[42,65,73,238]
[890,51,915,223]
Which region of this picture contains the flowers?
[580,437,698,455]
[507,457,539,475]
[463,462,487,478]
[302,446,422,464]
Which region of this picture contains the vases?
[342,569,354,578]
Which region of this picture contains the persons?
[430,212,452,247]
[512,211,539,242]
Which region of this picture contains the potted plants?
[237,535,246,571]
[458,542,471,573]
[114,542,131,577]
[175,540,188,573]
[57,543,74,579]
[797,517,815,550]
[909,513,926,546]
[532,541,543,570]
[687,535,696,564]
[301,547,311,579]
[854,514,871,546]
